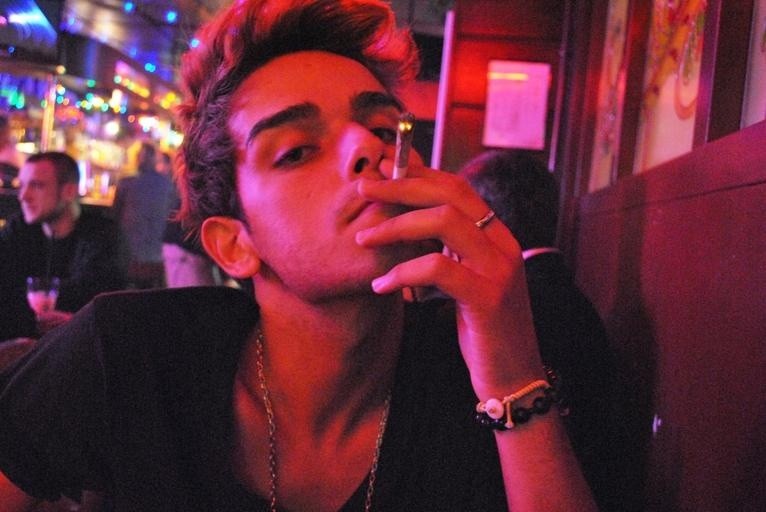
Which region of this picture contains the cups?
[24,276,63,315]
